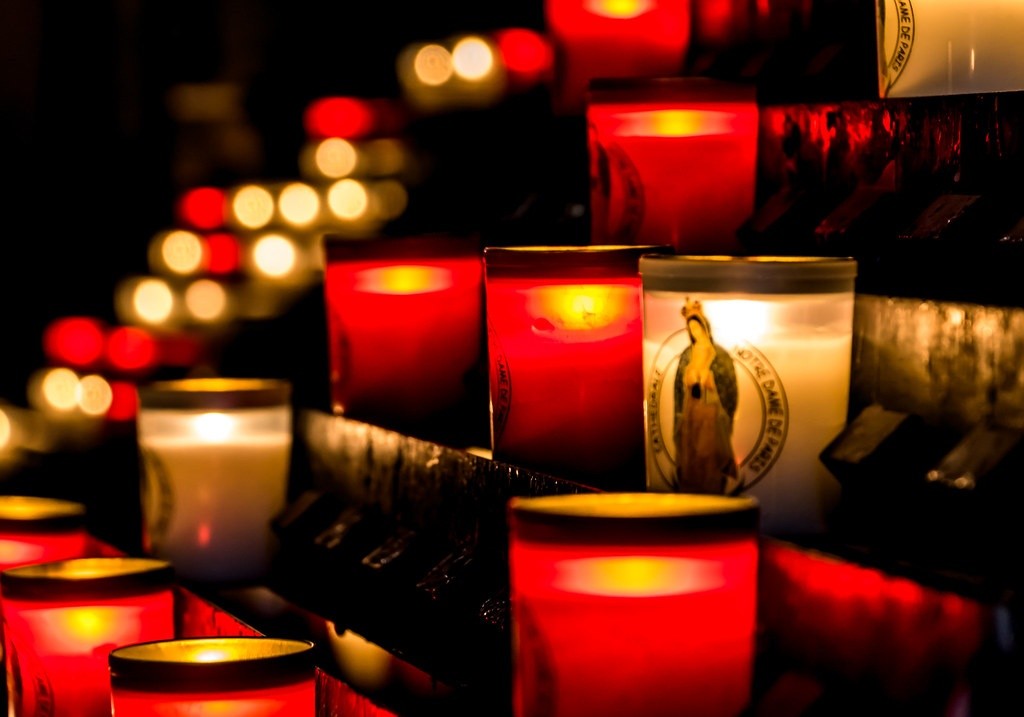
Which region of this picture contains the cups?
[874,0,1022,96]
[481,244,668,492]
[0,559,175,717]
[543,0,691,98]
[589,76,759,252]
[136,377,294,582]
[505,492,763,717]
[318,237,486,452]
[0,494,89,568]
[108,635,318,717]
[637,252,855,546]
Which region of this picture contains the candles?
[0,0,1024,717]
